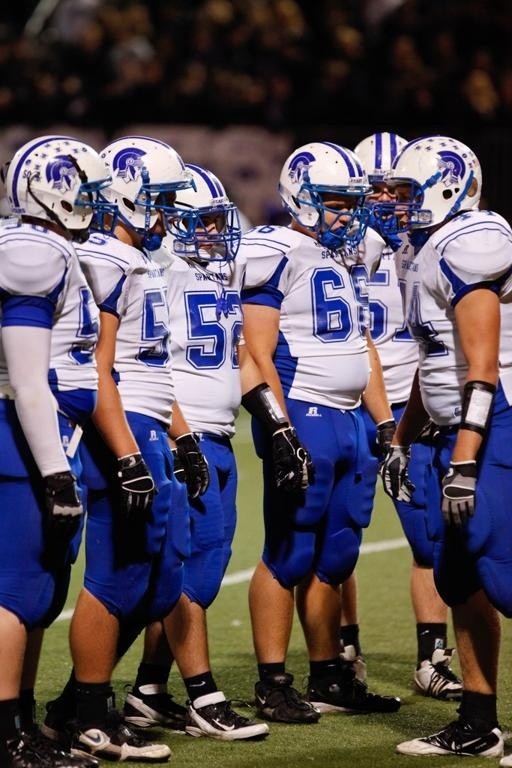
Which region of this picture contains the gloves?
[41,470,83,541]
[439,459,478,525]
[115,453,160,513]
[169,433,210,498]
[377,419,416,504]
[270,428,316,492]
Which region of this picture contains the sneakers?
[414,647,464,701]
[1,724,97,768]
[186,692,268,740]
[305,646,401,714]
[396,721,505,762]
[123,688,188,728]
[252,675,322,724]
[42,702,171,761]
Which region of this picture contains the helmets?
[5,135,116,237]
[387,137,482,228]
[176,163,240,261]
[100,135,189,239]
[354,132,410,183]
[278,142,372,247]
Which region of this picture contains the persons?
[123,163,315,741]
[39,136,210,762]
[241,142,400,723]
[379,136,512,766]
[0,136,113,768]
[339,129,462,704]
